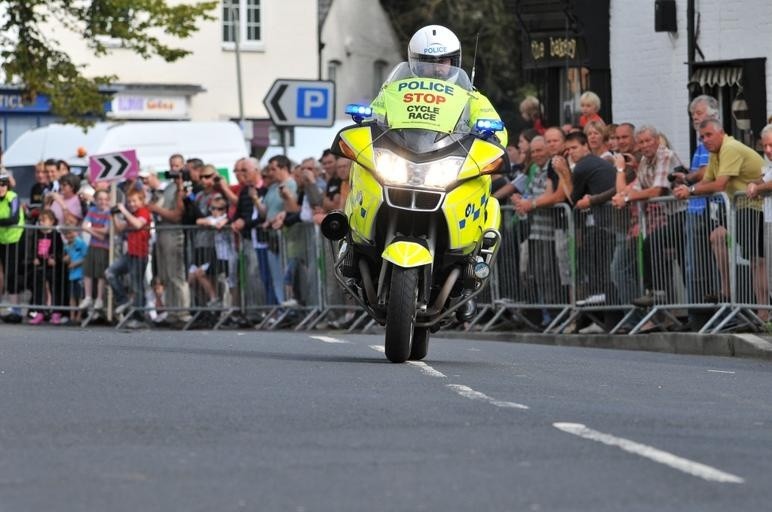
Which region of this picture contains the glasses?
[200,174,212,179]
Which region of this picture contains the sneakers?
[577,294,768,333]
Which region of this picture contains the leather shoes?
[456,289,477,321]
[344,277,358,294]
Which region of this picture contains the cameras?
[668,165,688,181]
[214,177,219,182]
[165,168,189,181]
[110,205,120,214]
[623,155,628,162]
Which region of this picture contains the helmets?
[407,25,461,84]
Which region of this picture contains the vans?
[0,117,250,207]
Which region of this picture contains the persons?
[492,94,772,335]
[363,26,508,153]
[0,153,354,329]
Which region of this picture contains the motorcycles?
[318,60,509,363]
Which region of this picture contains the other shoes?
[1,297,355,329]
